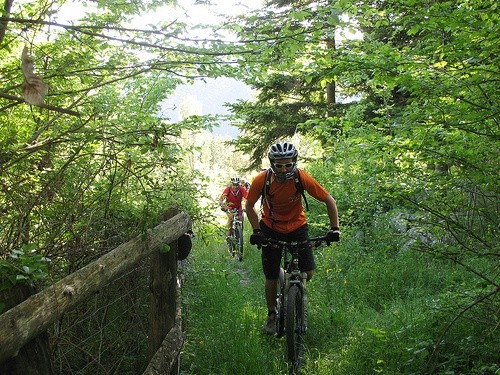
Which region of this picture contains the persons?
[245,143,339,336]
[219,177,250,242]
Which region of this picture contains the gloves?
[250,229,263,249]
[326,227,340,247]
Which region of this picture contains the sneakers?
[265,312,276,336]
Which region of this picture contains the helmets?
[268,142,298,178]
[230,177,240,184]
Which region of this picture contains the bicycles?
[221,208,246,260]
[251,230,340,365]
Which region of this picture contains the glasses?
[273,162,295,168]
